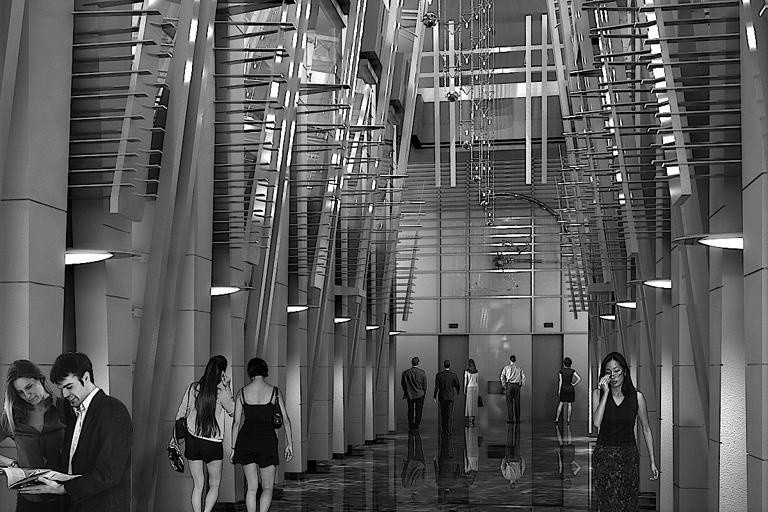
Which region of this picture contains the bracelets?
[231,447,234,449]
[11,460,18,467]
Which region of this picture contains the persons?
[400,431,426,501]
[0,360,77,512]
[500,422,527,489]
[552,357,581,424]
[401,356,427,433]
[433,360,460,436]
[432,435,461,497]
[553,423,581,489]
[168,354,235,512]
[228,357,294,511]
[463,423,481,487]
[463,358,480,427]
[500,355,525,423]
[17,351,134,511]
[592,352,659,512]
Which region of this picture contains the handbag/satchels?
[274,404,283,428]
[176,418,188,439]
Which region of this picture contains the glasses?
[609,368,624,379]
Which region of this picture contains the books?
[0,466,83,490]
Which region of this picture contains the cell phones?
[221,370,225,377]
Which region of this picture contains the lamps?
[333,316,360,324]
[603,301,637,309]
[388,331,405,336]
[286,304,320,313]
[672,233,743,250]
[365,325,386,331]
[210,285,256,296]
[626,279,671,289]
[64,249,141,266]
[590,314,616,321]
